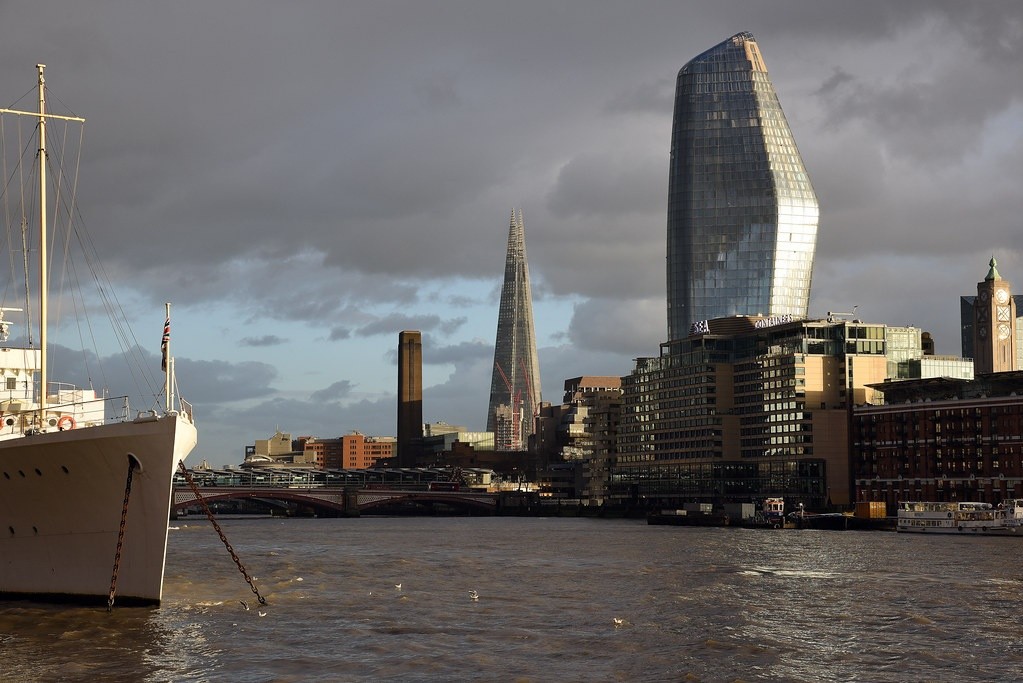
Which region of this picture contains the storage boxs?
[659,503,756,519]
[855,501,887,519]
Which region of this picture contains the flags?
[161,317,170,371]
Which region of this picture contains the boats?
[895,495,1023,537]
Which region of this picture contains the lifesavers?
[1010,509,1014,513]
[3,414,17,427]
[45,414,59,428]
[58,415,76,430]
[982,526,986,531]
[958,526,962,531]
[948,513,951,517]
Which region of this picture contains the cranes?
[516,357,541,435]
[495,362,523,451]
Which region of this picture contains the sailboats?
[0,59,197,612]
